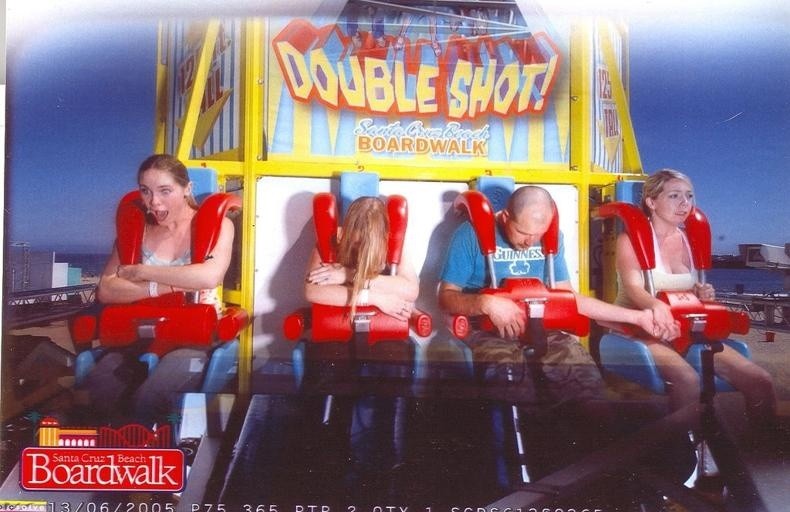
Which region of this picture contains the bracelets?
[171,286,174,293]
[360,288,368,307]
[150,281,158,298]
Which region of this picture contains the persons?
[304,195,420,499]
[85,153,235,512]
[603,168,790,506]
[434,185,608,481]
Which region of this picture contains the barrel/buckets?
[766,331,775,342]
